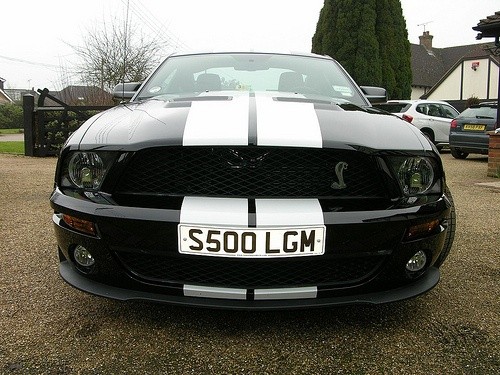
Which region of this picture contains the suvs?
[370,100,461,152]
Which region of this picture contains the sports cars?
[50,47,456,307]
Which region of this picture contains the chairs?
[278,70,307,95]
[195,72,225,92]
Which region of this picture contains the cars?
[449,100,500,158]
